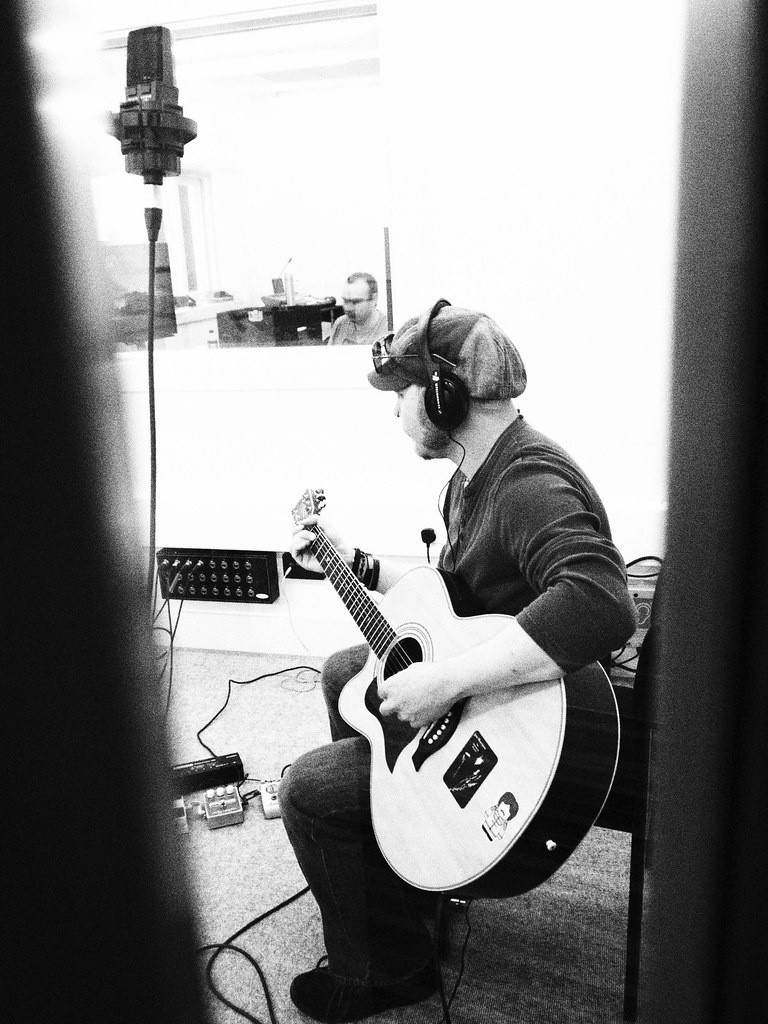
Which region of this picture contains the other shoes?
[291,967,440,1022]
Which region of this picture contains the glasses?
[372,334,456,373]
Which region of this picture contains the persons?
[327,272,391,346]
[280,306,640,1023]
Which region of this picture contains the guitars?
[290,490,623,899]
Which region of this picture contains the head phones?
[417,299,469,431]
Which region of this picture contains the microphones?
[311,296,337,310]
[109,26,197,176]
[420,528,436,563]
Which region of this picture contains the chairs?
[427,576,664,1024]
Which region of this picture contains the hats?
[366,306,529,402]
